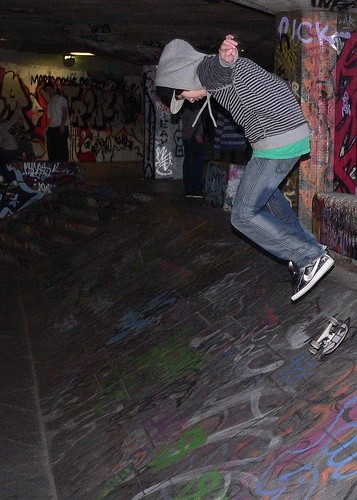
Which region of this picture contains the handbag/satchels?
[76,151,96,162]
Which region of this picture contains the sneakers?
[290,254,335,301]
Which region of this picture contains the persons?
[42,82,71,162]
[154,35,336,301]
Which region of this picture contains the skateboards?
[308,313,348,361]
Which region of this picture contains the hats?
[156,85,185,115]
